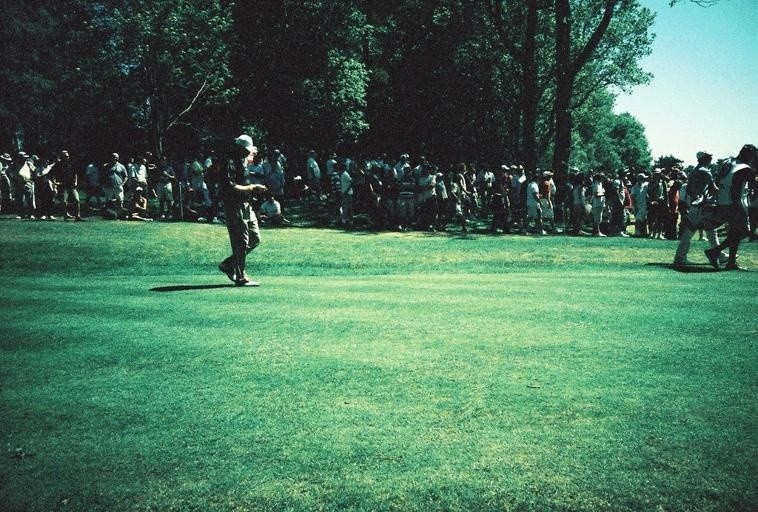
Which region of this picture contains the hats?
[18,151,30,158]
[235,134,259,154]
[63,150,69,157]
[293,149,555,183]
[570,166,687,189]
[32,154,40,161]
[0,152,13,162]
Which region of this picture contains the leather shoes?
[233,277,260,287]
[218,262,237,282]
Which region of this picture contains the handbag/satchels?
[684,194,729,232]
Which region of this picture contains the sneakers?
[491,220,709,242]
[704,245,749,272]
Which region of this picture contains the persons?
[219,134,268,287]
[701,142,757,271]
[673,149,739,271]
